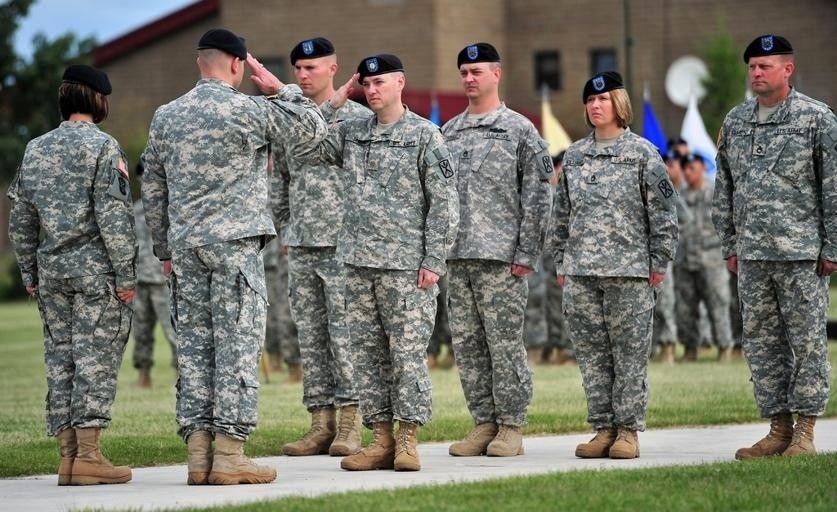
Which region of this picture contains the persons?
[7,63,139,485]
[545,71,679,459]
[129,159,179,388]
[440,43,554,456]
[713,34,837,461]
[288,54,459,471]
[426,297,455,371]
[270,37,375,457]
[260,237,303,384]
[142,28,328,486]
[532,149,572,364]
[653,136,734,366]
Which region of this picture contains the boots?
[285,364,302,385]
[268,353,284,374]
[782,415,817,457]
[610,428,640,459]
[442,353,455,368]
[57,428,78,486]
[449,422,498,456]
[72,428,132,485]
[341,424,396,471]
[575,428,615,458]
[137,368,152,388]
[283,407,337,455]
[736,411,795,461]
[330,407,361,456]
[187,430,215,485]
[426,354,439,370]
[681,347,698,363]
[209,433,276,485]
[394,421,421,471]
[732,349,742,360]
[718,346,734,362]
[653,344,673,364]
[487,425,524,456]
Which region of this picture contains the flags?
[681,92,718,185]
[642,81,671,158]
[540,87,571,155]
[429,89,441,125]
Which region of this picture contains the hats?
[582,71,625,104]
[291,37,335,66]
[662,150,681,161]
[457,43,501,69]
[667,137,686,149]
[356,55,404,85]
[743,34,794,64]
[61,66,112,96]
[197,29,247,60]
[681,153,703,169]
